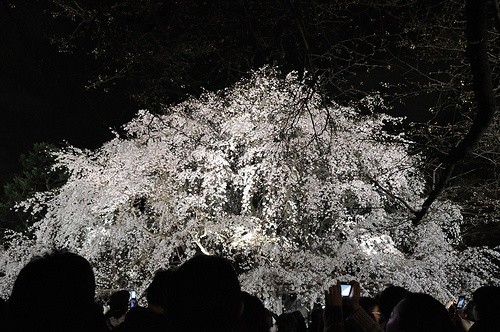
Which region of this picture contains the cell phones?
[130,298,136,308]
[339,282,353,297]
[457,296,465,310]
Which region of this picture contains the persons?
[114,268,173,332]
[242,275,500,332]
[99,290,132,332]
[155,255,242,331]
[1,250,99,332]
